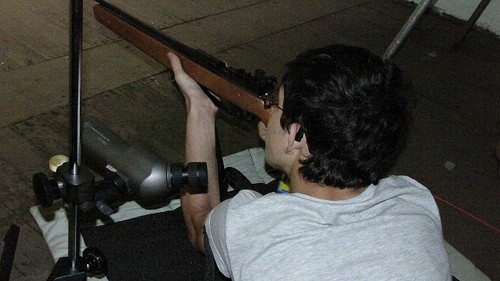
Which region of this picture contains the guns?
[89,3,277,120]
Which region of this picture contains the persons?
[167,43,452,281]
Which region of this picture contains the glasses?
[264,89,284,113]
